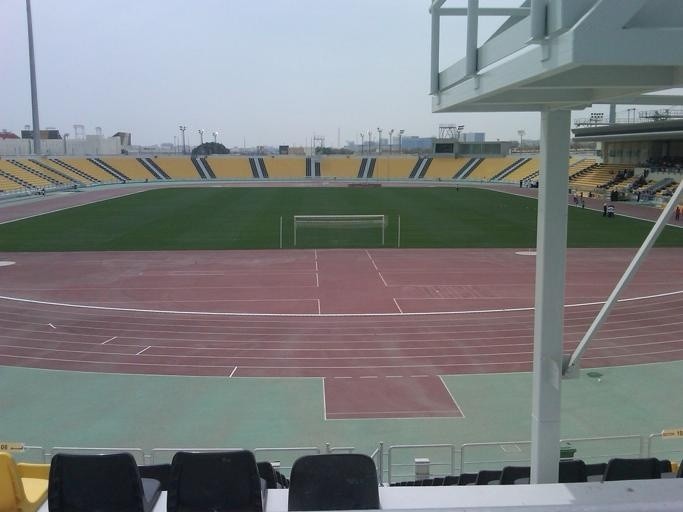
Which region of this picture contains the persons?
[581,201,584,208]
[575,200,578,206]
[639,154,682,178]
[675,206,680,221]
[617,169,627,178]
[573,195,577,206]
[601,203,607,216]
[607,205,614,217]
[518,179,538,190]
[610,190,619,201]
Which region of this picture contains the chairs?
[558,458,682,483]
[257,460,289,489]
[391,466,531,485]
[137,464,171,492]
[288,454,382,512]
[166,450,267,512]
[48,453,164,511]
[1,453,50,512]
